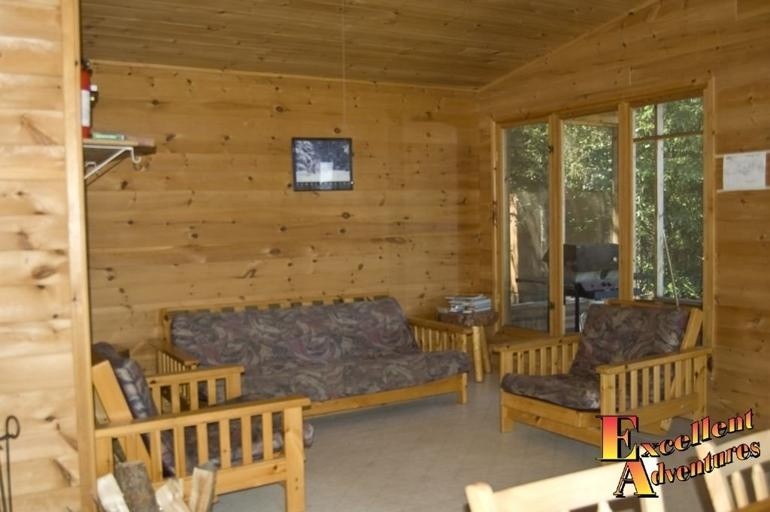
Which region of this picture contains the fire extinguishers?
[81,58,93,138]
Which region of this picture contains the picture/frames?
[291,137,354,192]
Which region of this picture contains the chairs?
[464,428,770,512]
[491,299,714,451]
[92,341,312,512]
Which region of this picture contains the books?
[443,291,492,315]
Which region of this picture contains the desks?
[436,309,499,383]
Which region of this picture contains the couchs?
[156,287,468,420]
